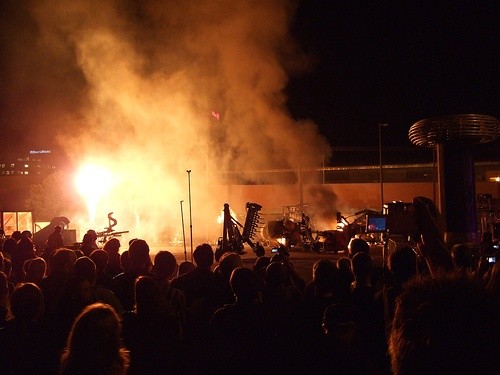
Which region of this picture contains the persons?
[0,224,500,375]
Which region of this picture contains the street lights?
[378,123,390,215]
